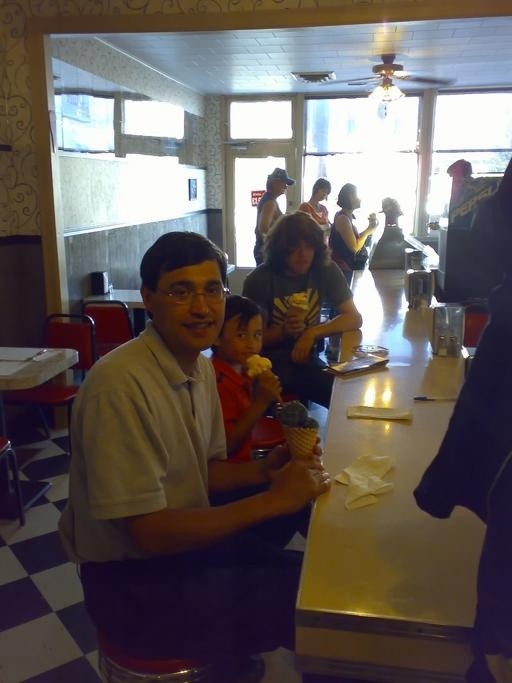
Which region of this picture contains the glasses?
[158,285,227,305]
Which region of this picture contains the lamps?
[366,77,411,120]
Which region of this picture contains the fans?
[317,54,456,87]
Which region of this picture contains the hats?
[270,168,295,185]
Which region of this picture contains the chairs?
[1,312,100,455]
[81,297,133,359]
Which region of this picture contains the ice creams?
[279,400,320,461]
[288,293,309,320]
[245,354,283,403]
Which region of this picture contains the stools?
[0,436,28,527]
[94,620,266,680]
[250,411,286,462]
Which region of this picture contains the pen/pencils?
[414,396,458,401]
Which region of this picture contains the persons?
[243,211,364,412]
[57,231,330,662]
[426,159,472,230]
[208,295,286,466]
[252,169,377,356]
